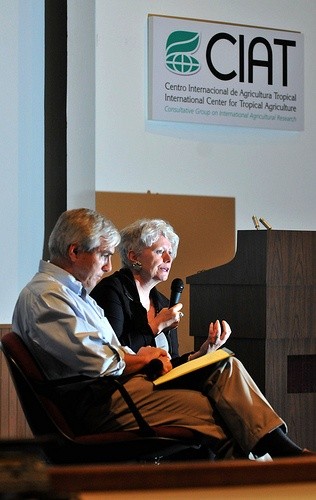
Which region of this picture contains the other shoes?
[299,448,316,456]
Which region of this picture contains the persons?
[89,218,232,369]
[11,208,316,462]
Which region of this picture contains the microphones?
[252,216,259,228]
[259,217,272,230]
[166,278,184,334]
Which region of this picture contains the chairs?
[0,332,201,466]
